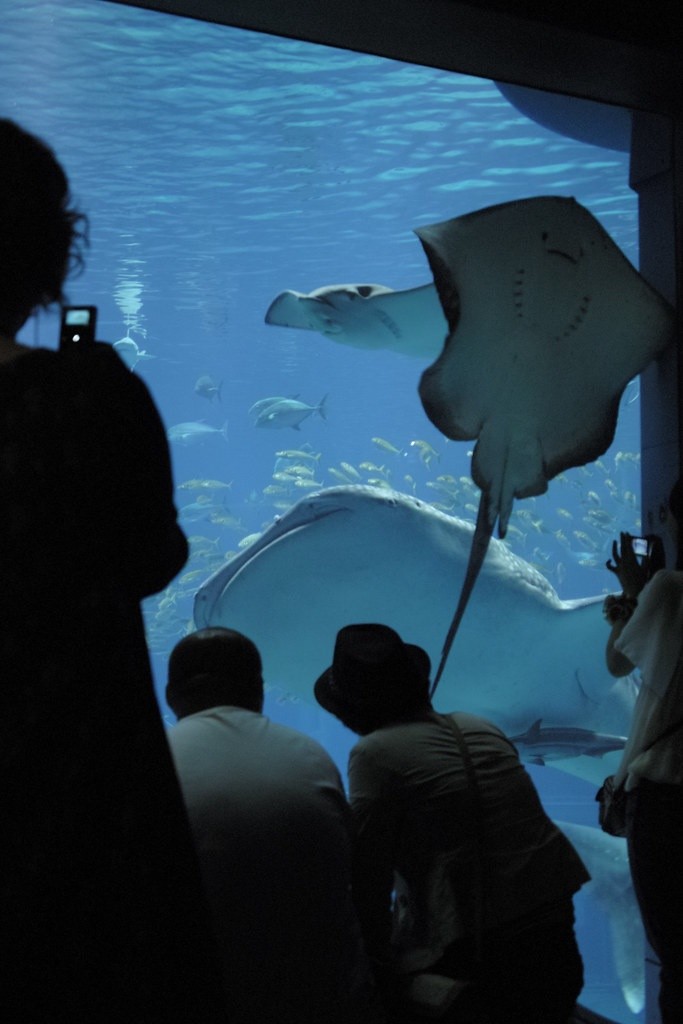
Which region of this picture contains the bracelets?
[604,593,637,623]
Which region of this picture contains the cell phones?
[630,537,648,557]
[59,305,95,354]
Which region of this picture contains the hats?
[314,624,431,722]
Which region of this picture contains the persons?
[315,621,588,1024]
[161,626,363,1022]
[605,473,683,1024]
[1,114,205,1024]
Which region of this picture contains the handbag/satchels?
[595,775,633,837]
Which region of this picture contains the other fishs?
[110,195,648,1018]
